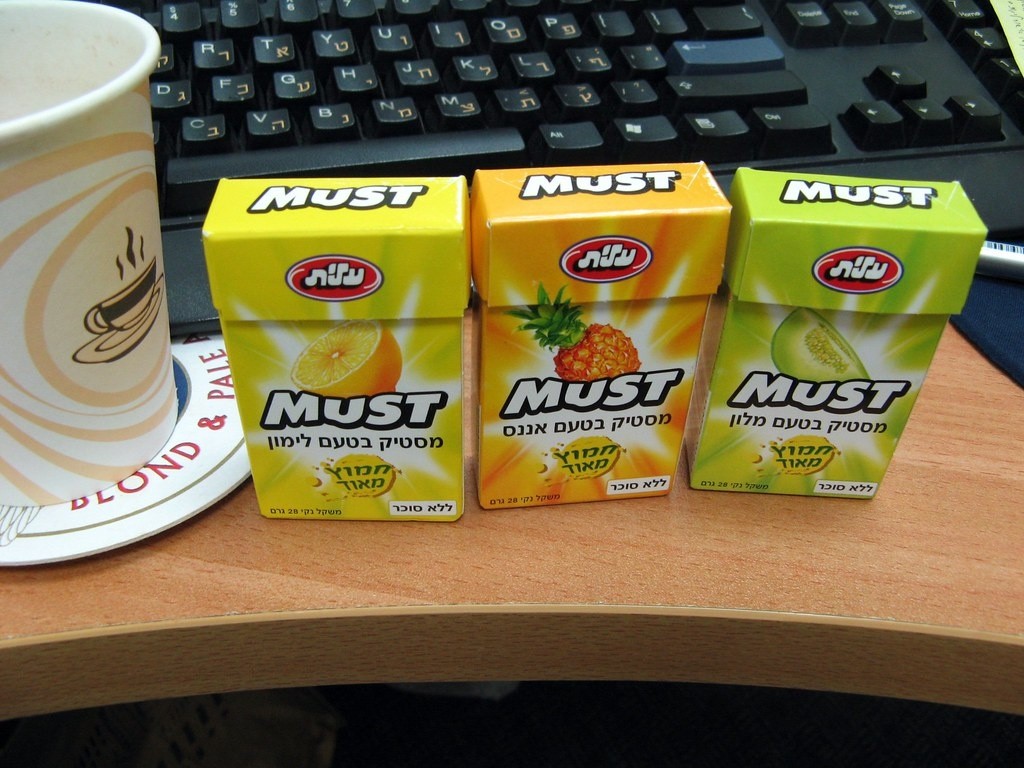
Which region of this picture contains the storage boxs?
[682,167,989,499]
[468,158,734,512]
[199,173,471,524]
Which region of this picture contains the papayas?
[771,306,871,382]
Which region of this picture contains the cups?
[0,0,179,507]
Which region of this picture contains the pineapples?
[505,281,641,380]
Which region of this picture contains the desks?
[0,319,1024,722]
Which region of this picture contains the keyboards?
[73,0,1024,338]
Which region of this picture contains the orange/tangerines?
[290,318,402,397]
